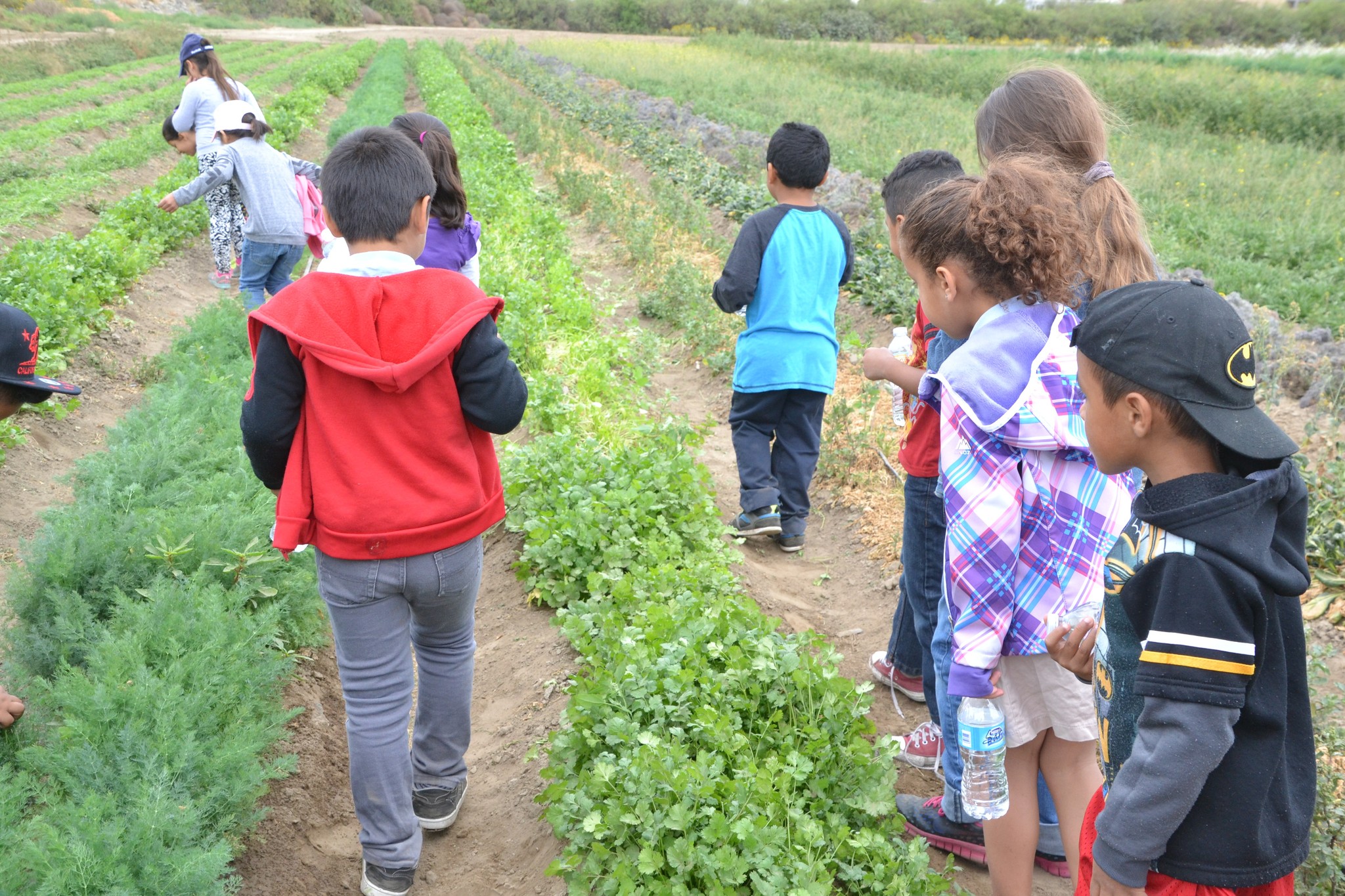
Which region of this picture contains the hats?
[210,100,257,143]
[1071,279,1301,460]
[0,302,82,395]
[178,34,214,77]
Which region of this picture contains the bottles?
[1047,601,1102,653]
[957,697,1010,820]
[888,327,911,426]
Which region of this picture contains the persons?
[0,302,81,731]
[859,65,1163,881]
[238,127,529,896]
[714,122,854,554]
[156,32,483,329]
[1070,275,1317,896]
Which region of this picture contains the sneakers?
[894,794,987,865]
[777,533,805,552]
[728,504,782,536]
[360,857,412,896]
[232,267,241,277]
[869,650,925,702]
[410,777,472,830]
[1033,850,1070,878]
[209,270,231,288]
[883,719,943,767]
